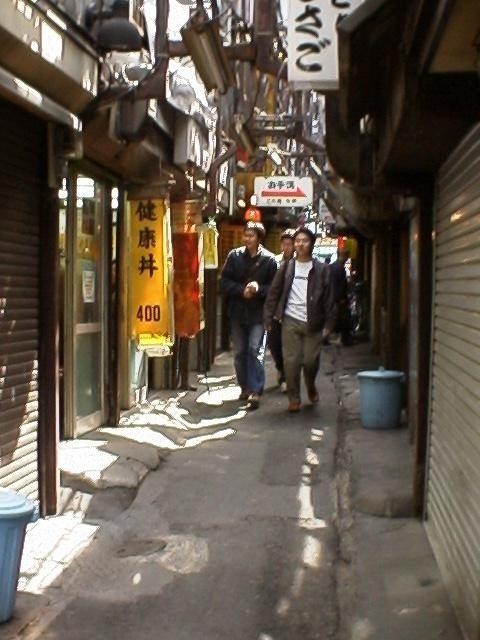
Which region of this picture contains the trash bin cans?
[356,366,405,430]
[0,490,38,623]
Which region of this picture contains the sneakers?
[287,400,300,411]
[308,390,318,402]
[238,385,248,400]
[280,383,288,392]
[275,369,281,381]
[247,393,259,409]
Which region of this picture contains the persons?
[266,227,297,393]
[220,221,277,408]
[320,252,357,346]
[261,226,339,413]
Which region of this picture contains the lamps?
[180,0,235,97]
[84,0,144,54]
[231,113,260,156]
